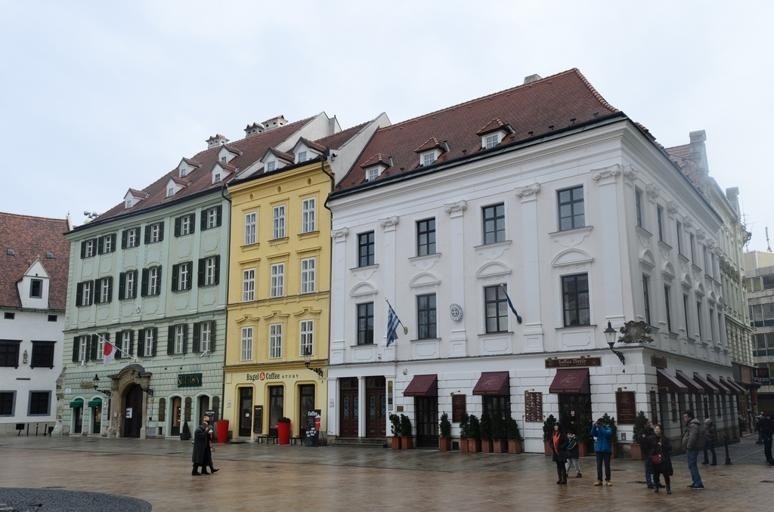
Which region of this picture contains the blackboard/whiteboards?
[305,408,321,443]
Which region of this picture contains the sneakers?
[576,473,581,478]
[593,479,603,486]
[648,484,671,494]
[560,478,567,484]
[557,480,561,483]
[605,480,613,486]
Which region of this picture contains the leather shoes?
[691,485,704,489]
[192,469,220,476]
[688,485,693,487]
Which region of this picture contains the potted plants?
[178,420,190,440]
[628,410,649,461]
[502,415,524,454]
[574,415,588,457]
[601,412,619,461]
[459,413,482,453]
[479,412,506,453]
[543,414,560,456]
[389,413,414,450]
[276,416,290,445]
[439,411,451,451]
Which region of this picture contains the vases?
[216,419,229,443]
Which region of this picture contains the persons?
[204,415,219,474]
[548,420,569,484]
[682,410,704,490]
[590,418,617,485]
[565,430,582,478]
[738,403,774,466]
[702,418,718,465]
[646,424,673,494]
[640,422,665,488]
[190,422,212,475]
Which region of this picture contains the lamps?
[92,373,111,397]
[603,319,625,365]
[302,347,323,377]
[23,350,28,363]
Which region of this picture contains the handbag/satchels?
[651,453,665,464]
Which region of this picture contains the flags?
[102,340,117,367]
[386,306,400,347]
